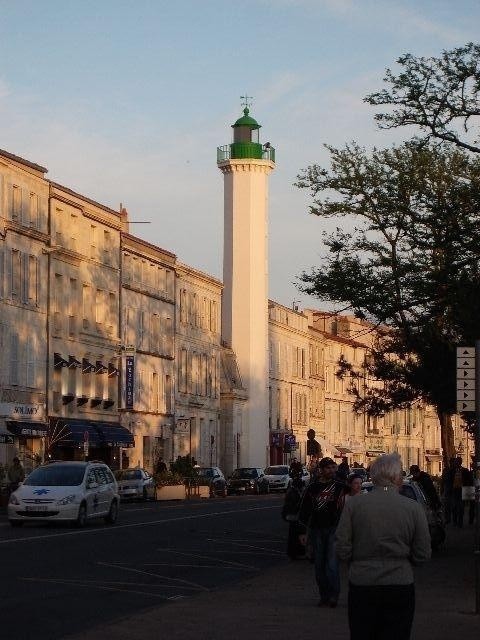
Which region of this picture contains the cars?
[188,465,365,496]
[353,480,448,546]
[115,469,156,500]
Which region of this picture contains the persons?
[7,457,24,486]
[155,457,168,474]
[280,456,479,640]
[122,452,129,469]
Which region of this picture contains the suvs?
[7,459,122,526]
